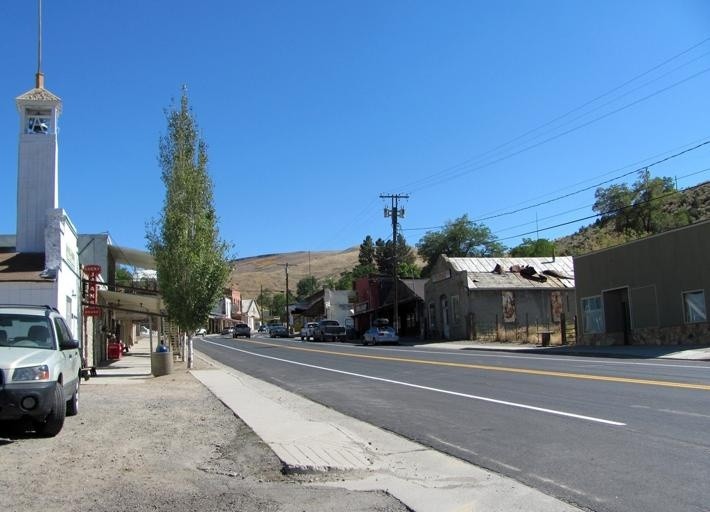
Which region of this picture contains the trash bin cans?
[151,344,174,377]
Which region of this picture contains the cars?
[362,327,400,345]
[0,304,82,437]
[223,323,251,338]
[258,323,289,338]
[195,328,207,337]
[300,322,319,341]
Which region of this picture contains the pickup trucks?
[313,320,347,342]
